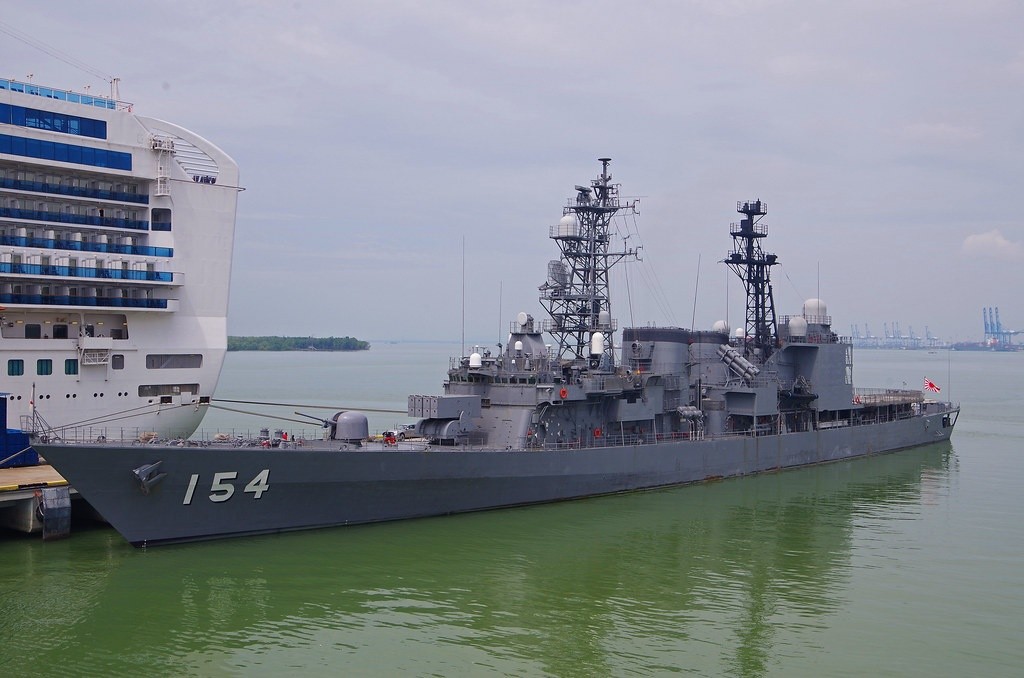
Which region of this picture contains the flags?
[924,377,941,393]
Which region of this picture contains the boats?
[20,155,962,551]
[2,18,244,440]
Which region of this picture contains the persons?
[390,432,396,444]
[282,432,287,441]
[290,435,295,441]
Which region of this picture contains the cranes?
[851,307,1024,354]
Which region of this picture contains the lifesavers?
[595,428,601,438]
[856,397,859,403]
[560,388,568,398]
